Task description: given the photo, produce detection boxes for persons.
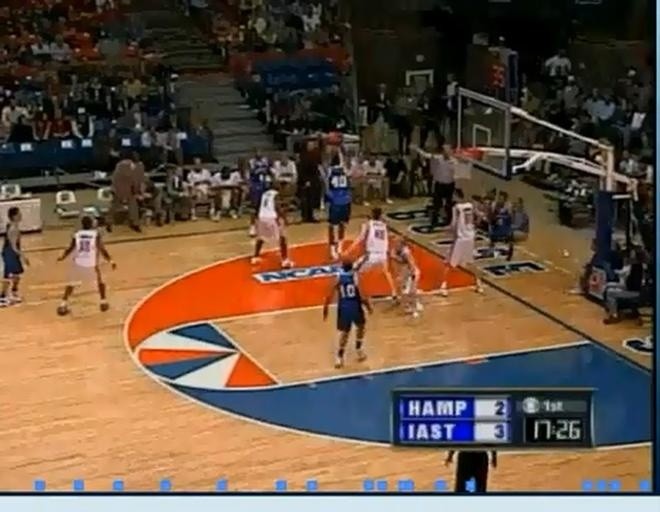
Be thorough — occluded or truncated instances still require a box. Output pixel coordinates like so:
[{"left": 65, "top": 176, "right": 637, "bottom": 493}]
[
  {"left": 0, "top": 0, "right": 657, "bottom": 369},
  {"left": 444, "top": 448, "right": 496, "bottom": 492}
]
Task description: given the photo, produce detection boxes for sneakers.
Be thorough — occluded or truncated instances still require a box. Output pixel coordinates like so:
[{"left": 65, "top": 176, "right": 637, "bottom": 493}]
[
  {"left": 362, "top": 200, "right": 370, "bottom": 207},
  {"left": 335, "top": 356, "right": 344, "bottom": 368},
  {"left": 571, "top": 287, "right": 586, "bottom": 294},
  {"left": 251, "top": 257, "right": 263, "bottom": 264},
  {"left": 470, "top": 284, "right": 484, "bottom": 293},
  {"left": 282, "top": 257, "right": 295, "bottom": 268},
  {"left": 329, "top": 249, "right": 343, "bottom": 259},
  {"left": 107, "top": 208, "right": 239, "bottom": 232},
  {"left": 603, "top": 314, "right": 621, "bottom": 325},
  {"left": 433, "top": 288, "right": 449, "bottom": 296},
  {"left": 249, "top": 225, "right": 257, "bottom": 236},
  {"left": 57, "top": 306, "right": 66, "bottom": 315},
  {"left": 0, "top": 294, "right": 22, "bottom": 306},
  {"left": 386, "top": 198, "right": 393, "bottom": 204},
  {"left": 100, "top": 302, "right": 110, "bottom": 310},
  {"left": 357, "top": 349, "right": 368, "bottom": 362}
]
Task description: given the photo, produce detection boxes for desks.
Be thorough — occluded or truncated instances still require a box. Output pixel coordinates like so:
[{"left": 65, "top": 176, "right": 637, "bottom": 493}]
[{"left": 0, "top": 198, "right": 41, "bottom": 234}]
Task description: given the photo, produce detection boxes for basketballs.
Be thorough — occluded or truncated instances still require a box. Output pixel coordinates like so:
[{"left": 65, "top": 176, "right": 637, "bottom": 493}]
[{"left": 328, "top": 133, "right": 342, "bottom": 145}]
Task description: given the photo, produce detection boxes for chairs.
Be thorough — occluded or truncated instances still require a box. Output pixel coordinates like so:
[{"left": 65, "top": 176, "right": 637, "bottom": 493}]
[
  {"left": 0, "top": 184, "right": 21, "bottom": 195},
  {"left": 53, "top": 190, "right": 80, "bottom": 230},
  {"left": 96, "top": 187, "right": 113, "bottom": 213}
]
